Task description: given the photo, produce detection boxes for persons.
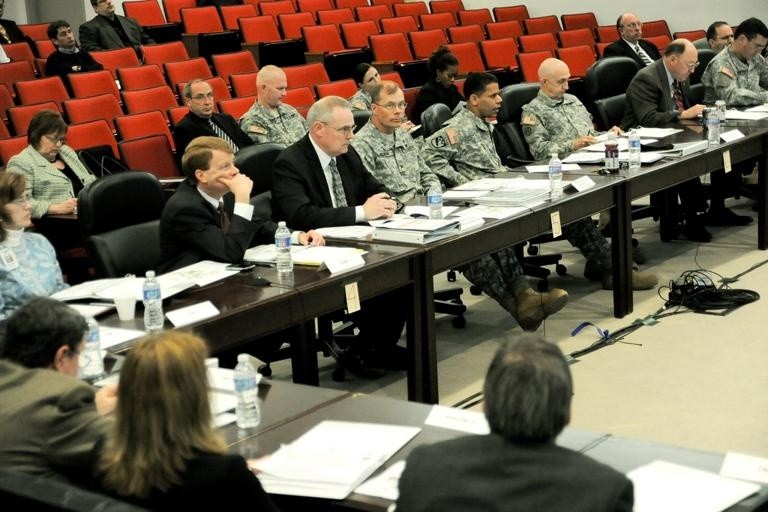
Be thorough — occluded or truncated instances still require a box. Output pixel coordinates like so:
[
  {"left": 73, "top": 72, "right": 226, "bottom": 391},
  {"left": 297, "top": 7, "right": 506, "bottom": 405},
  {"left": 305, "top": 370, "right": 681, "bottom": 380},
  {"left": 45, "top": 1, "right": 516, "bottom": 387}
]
[
  {"left": 0, "top": 1, "right": 24, "bottom": 64},
  {"left": 0, "top": 171, "right": 68, "bottom": 323},
  {"left": 411, "top": 47, "right": 464, "bottom": 124},
  {"left": 520, "top": 58, "right": 648, "bottom": 265},
  {"left": 172, "top": 79, "right": 256, "bottom": 176},
  {"left": 44, "top": 21, "right": 104, "bottom": 78},
  {"left": 158, "top": 136, "right": 325, "bottom": 276},
  {"left": 706, "top": 21, "right": 733, "bottom": 51},
  {"left": 351, "top": 80, "right": 568, "bottom": 333},
  {"left": 0, "top": 297, "right": 117, "bottom": 487},
  {"left": 92, "top": 332, "right": 278, "bottom": 512},
  {"left": 419, "top": 70, "right": 658, "bottom": 291},
  {"left": 4, "top": 111, "right": 98, "bottom": 220},
  {"left": 619, "top": 39, "right": 713, "bottom": 243},
  {"left": 603, "top": 13, "right": 662, "bottom": 69},
  {"left": 394, "top": 335, "right": 634, "bottom": 512},
  {"left": 701, "top": 18, "right": 768, "bottom": 107},
  {"left": 269, "top": 95, "right": 407, "bottom": 380},
  {"left": 79, "top": 0, "right": 157, "bottom": 51},
  {"left": 349, "top": 63, "right": 416, "bottom": 134},
  {"left": 237, "top": 65, "right": 308, "bottom": 149}
]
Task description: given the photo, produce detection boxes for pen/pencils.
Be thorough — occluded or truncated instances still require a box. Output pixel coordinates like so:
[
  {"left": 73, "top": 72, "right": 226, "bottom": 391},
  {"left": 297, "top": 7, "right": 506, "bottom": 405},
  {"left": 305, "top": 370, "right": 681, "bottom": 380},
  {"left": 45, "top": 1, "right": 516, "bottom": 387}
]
[
  {"left": 252, "top": 262, "right": 276, "bottom": 269},
  {"left": 586, "top": 129, "right": 591, "bottom": 137},
  {"left": 68, "top": 190, "right": 74, "bottom": 199},
  {"left": 382, "top": 196, "right": 401, "bottom": 200}
]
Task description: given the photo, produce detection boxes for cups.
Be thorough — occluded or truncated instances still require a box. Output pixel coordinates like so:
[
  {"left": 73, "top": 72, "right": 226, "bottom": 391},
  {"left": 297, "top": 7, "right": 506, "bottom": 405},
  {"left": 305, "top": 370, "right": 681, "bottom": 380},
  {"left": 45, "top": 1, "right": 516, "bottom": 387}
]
[{"left": 111, "top": 292, "right": 137, "bottom": 321}]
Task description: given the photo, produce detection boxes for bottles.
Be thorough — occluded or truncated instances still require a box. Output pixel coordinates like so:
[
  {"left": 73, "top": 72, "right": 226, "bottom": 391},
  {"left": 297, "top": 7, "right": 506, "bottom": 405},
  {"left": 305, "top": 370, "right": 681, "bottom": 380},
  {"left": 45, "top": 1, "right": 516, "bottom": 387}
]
[
  {"left": 628, "top": 129, "right": 641, "bottom": 168},
  {"left": 708, "top": 108, "right": 720, "bottom": 145},
  {"left": 548, "top": 153, "right": 563, "bottom": 197},
  {"left": 143, "top": 271, "right": 164, "bottom": 329},
  {"left": 604, "top": 143, "right": 619, "bottom": 173},
  {"left": 702, "top": 108, "right": 711, "bottom": 129},
  {"left": 80, "top": 317, "right": 104, "bottom": 375},
  {"left": 274, "top": 222, "right": 294, "bottom": 272},
  {"left": 715, "top": 100, "right": 726, "bottom": 122},
  {"left": 233, "top": 354, "right": 261, "bottom": 429},
  {"left": 426, "top": 182, "right": 443, "bottom": 220}
]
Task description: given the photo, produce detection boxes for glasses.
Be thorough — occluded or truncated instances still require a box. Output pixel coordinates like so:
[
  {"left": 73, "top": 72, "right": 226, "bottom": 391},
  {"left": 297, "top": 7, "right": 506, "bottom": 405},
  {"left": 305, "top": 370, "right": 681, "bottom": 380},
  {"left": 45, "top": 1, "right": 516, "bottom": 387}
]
[
  {"left": 43, "top": 134, "right": 67, "bottom": 145},
  {"left": 619, "top": 22, "right": 644, "bottom": 29},
  {"left": 191, "top": 93, "right": 214, "bottom": 100},
  {"left": 7, "top": 196, "right": 35, "bottom": 206},
  {"left": 373, "top": 101, "right": 407, "bottom": 110},
  {"left": 675, "top": 59, "right": 700, "bottom": 68},
  {"left": 321, "top": 121, "right": 357, "bottom": 135}
]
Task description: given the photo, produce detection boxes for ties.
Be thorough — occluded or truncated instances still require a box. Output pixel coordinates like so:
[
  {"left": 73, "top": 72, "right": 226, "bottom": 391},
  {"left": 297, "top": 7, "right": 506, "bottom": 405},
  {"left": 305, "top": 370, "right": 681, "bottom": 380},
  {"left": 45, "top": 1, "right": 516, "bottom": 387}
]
[
  {"left": 633, "top": 45, "right": 652, "bottom": 66},
  {"left": 205, "top": 117, "right": 240, "bottom": 156},
  {"left": 671, "top": 82, "right": 686, "bottom": 114},
  {"left": 329, "top": 160, "right": 350, "bottom": 210},
  {"left": 217, "top": 201, "right": 231, "bottom": 235}
]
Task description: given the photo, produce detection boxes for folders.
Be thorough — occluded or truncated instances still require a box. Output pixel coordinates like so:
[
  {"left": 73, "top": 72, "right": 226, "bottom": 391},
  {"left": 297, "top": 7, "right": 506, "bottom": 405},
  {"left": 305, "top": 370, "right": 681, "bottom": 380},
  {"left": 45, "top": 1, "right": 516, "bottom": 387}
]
[
  {"left": 471, "top": 186, "right": 552, "bottom": 207},
  {"left": 372, "top": 229, "right": 462, "bottom": 247},
  {"left": 97, "top": 325, "right": 147, "bottom": 355},
  {"left": 656, "top": 139, "right": 710, "bottom": 158},
  {"left": 375, "top": 219, "right": 462, "bottom": 234}
]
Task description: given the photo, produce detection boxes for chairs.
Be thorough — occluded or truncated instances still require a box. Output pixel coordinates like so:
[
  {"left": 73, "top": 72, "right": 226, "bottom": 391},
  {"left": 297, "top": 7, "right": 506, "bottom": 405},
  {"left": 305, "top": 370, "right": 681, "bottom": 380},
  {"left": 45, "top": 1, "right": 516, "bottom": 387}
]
[
  {"left": 493, "top": 5, "right": 530, "bottom": 31},
  {"left": 0, "top": 59, "right": 35, "bottom": 96},
  {"left": 523, "top": 15, "right": 562, "bottom": 44},
  {"left": 421, "top": 102, "right": 567, "bottom": 295},
  {"left": 0, "top": 134, "right": 31, "bottom": 163},
  {"left": 583, "top": 57, "right": 660, "bottom": 221},
  {"left": 161, "top": 0, "right": 196, "bottom": 24},
  {"left": 5, "top": 98, "right": 62, "bottom": 136},
  {"left": 122, "top": 0, "right": 176, "bottom": 32},
  {"left": 277, "top": 11, "right": 317, "bottom": 41},
  {"left": 162, "top": 56, "right": 231, "bottom": 95},
  {"left": 408, "top": 28, "right": 448, "bottom": 63},
  {"left": 116, "top": 64, "right": 178, "bottom": 100},
  {"left": 517, "top": 32, "right": 557, "bottom": 58},
  {"left": 220, "top": 3, "right": 258, "bottom": 31},
  {"left": 380, "top": 72, "right": 404, "bottom": 88},
  {"left": 484, "top": 20, "right": 524, "bottom": 47},
  {"left": 447, "top": 24, "right": 486, "bottom": 52},
  {"left": 498, "top": 82, "right": 639, "bottom": 255},
  {"left": 392, "top": 2, "right": 429, "bottom": 27},
  {"left": 33, "top": 57, "right": 50, "bottom": 76},
  {"left": 515, "top": 49, "right": 555, "bottom": 83},
  {"left": 2, "top": 42, "right": 37, "bottom": 72},
  {"left": 279, "top": 62, "right": 330, "bottom": 99},
  {"left": 368, "top": 32, "right": 425, "bottom": 68},
  {"left": 343, "top": 111, "right": 466, "bottom": 336},
  {"left": 335, "top": 0, "right": 369, "bottom": 18},
  {"left": 12, "top": 75, "right": 70, "bottom": 113},
  {"left": 113, "top": 109, "right": 178, "bottom": 155},
  {"left": 225, "top": 141, "right": 366, "bottom": 377},
  {"left": 60, "top": 92, "right": 124, "bottom": 136},
  {"left": 480, "top": 37, "right": 519, "bottom": 72},
  {"left": 371, "top": 0, "right": 404, "bottom": 17},
  {"left": 445, "top": 41, "right": 503, "bottom": 77},
  {"left": 340, "top": 19, "right": 380, "bottom": 51},
  {"left": 641, "top": 20, "right": 673, "bottom": 41},
  {"left": 429, "top": 0, "right": 465, "bottom": 25},
  {"left": 117, "top": 132, "right": 186, "bottom": 190},
  {"left": 167, "top": 107, "right": 189, "bottom": 128},
  {"left": 18, "top": 21, "right": 50, "bottom": 41},
  {"left": 417, "top": 11, "right": 457, "bottom": 42},
  {"left": 78, "top": 171, "right": 165, "bottom": 280},
  {"left": 297, "top": 0, "right": 333, "bottom": 22},
  {"left": 315, "top": 7, "right": 355, "bottom": 34},
  {"left": 594, "top": 25, "right": 621, "bottom": 42},
  {"left": 314, "top": 78, "right": 358, "bottom": 103},
  {"left": 237, "top": 14, "right": 297, "bottom": 47},
  {"left": 67, "top": 68, "right": 123, "bottom": 105},
  {"left": 0, "top": 83, "right": 16, "bottom": 123},
  {"left": 594, "top": 42, "right": 613, "bottom": 59},
  {"left": 229, "top": 71, "right": 258, "bottom": 99},
  {"left": 457, "top": 8, "right": 494, "bottom": 35},
  {"left": 687, "top": 49, "right": 759, "bottom": 212},
  {"left": 35, "top": 39, "right": 57, "bottom": 59},
  {"left": 673, "top": 30, "right": 706, "bottom": 43},
  {"left": 139, "top": 40, "right": 190, "bottom": 78},
  {"left": 179, "top": 5, "right": 234, "bottom": 37},
  {"left": 301, "top": 22, "right": 364, "bottom": 56},
  {"left": 642, "top": 35, "right": 670, "bottom": 56},
  {"left": 88, "top": 47, "right": 140, "bottom": 82},
  {"left": 60, "top": 118, "right": 121, "bottom": 161},
  {"left": 216, "top": 93, "right": 257, "bottom": 119},
  {"left": 258, "top": 0, "right": 296, "bottom": 25},
  {"left": 281, "top": 86, "right": 316, "bottom": 118},
  {"left": 175, "top": 76, "right": 232, "bottom": 115},
  {"left": 379, "top": 14, "right": 418, "bottom": 44},
  {"left": 402, "top": 89, "right": 420, "bottom": 116},
  {"left": 557, "top": 45, "right": 596, "bottom": 78},
  {"left": 354, "top": 4, "right": 392, "bottom": 33},
  {"left": 556, "top": 28, "right": 597, "bottom": 58},
  {"left": 561, "top": 12, "right": 598, "bottom": 40},
  {"left": 211, "top": 49, "right": 259, "bottom": 88},
  {"left": 120, "top": 83, "right": 179, "bottom": 126}
]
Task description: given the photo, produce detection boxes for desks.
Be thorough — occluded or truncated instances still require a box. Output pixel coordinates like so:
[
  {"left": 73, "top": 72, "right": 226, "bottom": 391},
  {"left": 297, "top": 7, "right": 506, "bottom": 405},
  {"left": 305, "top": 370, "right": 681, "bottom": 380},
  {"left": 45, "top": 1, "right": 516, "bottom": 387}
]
[
  {"left": 663, "top": 103, "right": 768, "bottom": 128},
  {"left": 103, "top": 353, "right": 351, "bottom": 452},
  {"left": 95, "top": 239, "right": 424, "bottom": 404},
  {"left": 357, "top": 172, "right": 628, "bottom": 404},
  {"left": 507, "top": 125, "right": 768, "bottom": 314},
  {"left": 222, "top": 392, "right": 768, "bottom": 512}
]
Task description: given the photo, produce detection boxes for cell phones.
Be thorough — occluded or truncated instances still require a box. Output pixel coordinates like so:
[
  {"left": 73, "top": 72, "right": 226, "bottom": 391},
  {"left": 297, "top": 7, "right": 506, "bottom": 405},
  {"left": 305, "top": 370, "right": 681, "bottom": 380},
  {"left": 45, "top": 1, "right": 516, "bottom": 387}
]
[{"left": 226, "top": 262, "right": 255, "bottom": 269}]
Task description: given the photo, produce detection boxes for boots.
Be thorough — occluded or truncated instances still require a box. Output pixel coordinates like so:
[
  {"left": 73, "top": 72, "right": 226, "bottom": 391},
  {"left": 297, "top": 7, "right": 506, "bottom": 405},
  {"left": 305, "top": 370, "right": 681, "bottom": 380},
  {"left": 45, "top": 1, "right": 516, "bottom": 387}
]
[
  {"left": 499, "top": 295, "right": 542, "bottom": 333},
  {"left": 507, "top": 276, "right": 569, "bottom": 329},
  {"left": 603, "top": 268, "right": 659, "bottom": 290}
]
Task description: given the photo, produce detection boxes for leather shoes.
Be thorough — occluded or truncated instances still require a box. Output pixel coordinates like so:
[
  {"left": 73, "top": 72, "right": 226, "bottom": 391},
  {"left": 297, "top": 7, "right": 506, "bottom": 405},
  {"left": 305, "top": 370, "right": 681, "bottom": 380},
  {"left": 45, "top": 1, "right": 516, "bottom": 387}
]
[
  {"left": 706, "top": 207, "right": 754, "bottom": 227},
  {"left": 678, "top": 215, "right": 713, "bottom": 243}
]
